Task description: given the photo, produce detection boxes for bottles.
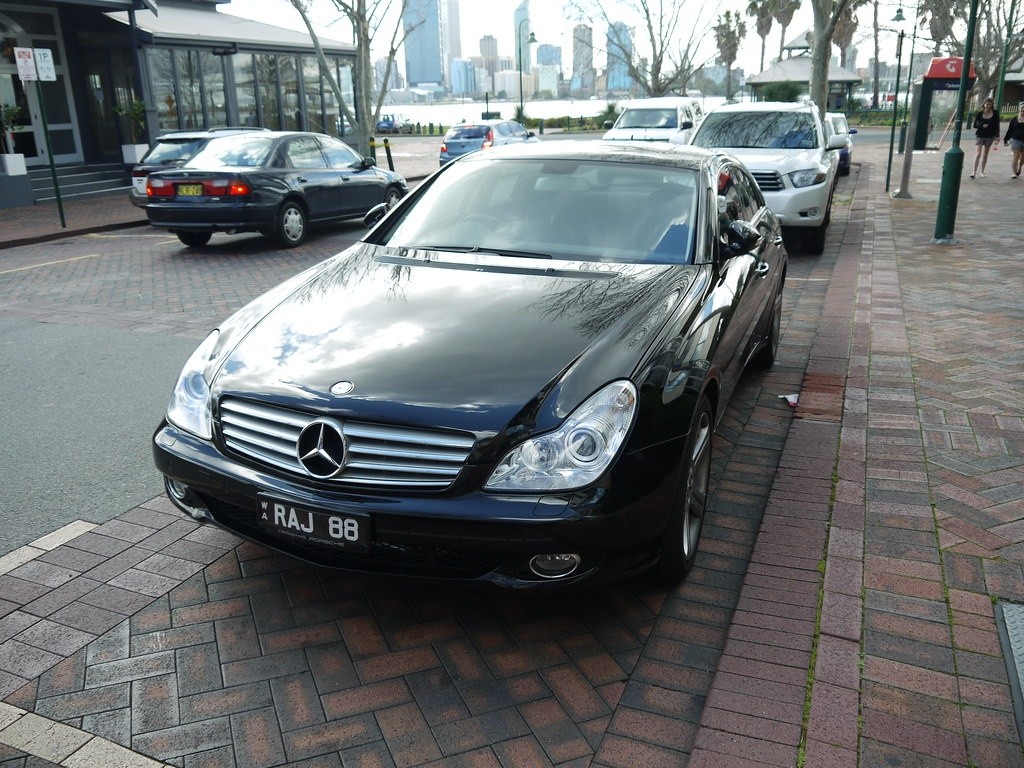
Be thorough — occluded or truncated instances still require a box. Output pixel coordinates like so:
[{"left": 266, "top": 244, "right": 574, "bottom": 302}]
[{"left": 994, "top": 141, "right": 998, "bottom": 152}]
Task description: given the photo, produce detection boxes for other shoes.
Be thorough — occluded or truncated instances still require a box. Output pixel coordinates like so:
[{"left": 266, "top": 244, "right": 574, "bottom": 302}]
[
  {"left": 980, "top": 173, "right": 987, "bottom": 177},
  {"left": 1017, "top": 171, "right": 1021, "bottom": 176},
  {"left": 1011, "top": 174, "right": 1017, "bottom": 178},
  {"left": 970, "top": 175, "right": 975, "bottom": 179}
]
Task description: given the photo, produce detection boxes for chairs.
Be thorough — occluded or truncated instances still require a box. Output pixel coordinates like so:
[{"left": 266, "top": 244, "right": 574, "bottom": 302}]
[
  {"left": 627, "top": 184, "right": 694, "bottom": 254},
  {"left": 487, "top": 190, "right": 573, "bottom": 245}
]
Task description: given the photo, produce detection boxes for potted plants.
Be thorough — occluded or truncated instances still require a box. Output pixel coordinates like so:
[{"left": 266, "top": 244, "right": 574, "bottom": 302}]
[
  {"left": 111, "top": 96, "right": 150, "bottom": 165},
  {"left": 0, "top": 104, "right": 31, "bottom": 176}
]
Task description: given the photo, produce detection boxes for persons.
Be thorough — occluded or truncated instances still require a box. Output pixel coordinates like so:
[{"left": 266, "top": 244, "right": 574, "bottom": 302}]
[
  {"left": 1003, "top": 102, "right": 1024, "bottom": 178},
  {"left": 970, "top": 98, "right": 1001, "bottom": 179}
]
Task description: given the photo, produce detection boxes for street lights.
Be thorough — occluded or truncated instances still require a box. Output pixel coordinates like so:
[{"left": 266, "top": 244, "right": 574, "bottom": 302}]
[
  {"left": 891, "top": 0, "right": 921, "bottom": 156},
  {"left": 519, "top": 18, "right": 539, "bottom": 125}
]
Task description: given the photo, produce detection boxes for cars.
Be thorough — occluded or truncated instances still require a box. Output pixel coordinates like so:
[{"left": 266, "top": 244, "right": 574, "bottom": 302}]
[
  {"left": 823, "top": 111, "right": 859, "bottom": 175},
  {"left": 128, "top": 127, "right": 273, "bottom": 208},
  {"left": 376, "top": 113, "right": 414, "bottom": 134},
  {"left": 439, "top": 120, "right": 542, "bottom": 168},
  {"left": 146, "top": 130, "right": 411, "bottom": 249},
  {"left": 152, "top": 139, "right": 790, "bottom": 599}
]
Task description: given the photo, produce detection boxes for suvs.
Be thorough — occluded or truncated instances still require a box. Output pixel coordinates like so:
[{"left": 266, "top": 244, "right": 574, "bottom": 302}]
[
  {"left": 684, "top": 100, "right": 849, "bottom": 256},
  {"left": 602, "top": 98, "right": 704, "bottom": 148}
]
